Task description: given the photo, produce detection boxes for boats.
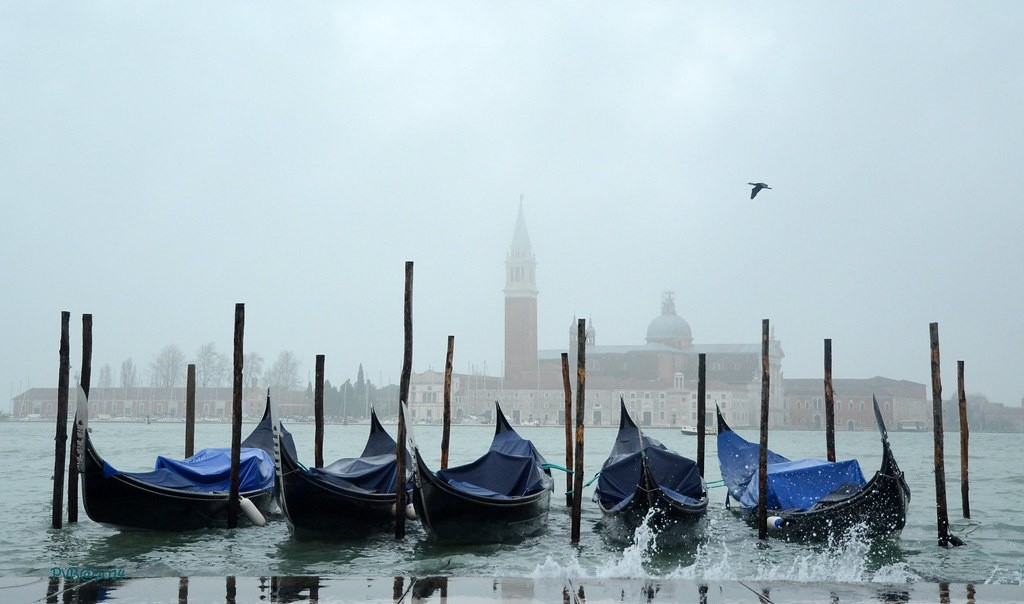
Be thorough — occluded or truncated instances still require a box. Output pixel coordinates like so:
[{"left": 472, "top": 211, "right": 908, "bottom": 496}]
[
  {"left": 715, "top": 405, "right": 911, "bottom": 545},
  {"left": 595, "top": 396, "right": 708, "bottom": 527},
  {"left": 75, "top": 379, "right": 279, "bottom": 531},
  {"left": 270, "top": 389, "right": 414, "bottom": 534},
  {"left": 681, "top": 424, "right": 716, "bottom": 437},
  {"left": 397, "top": 400, "right": 555, "bottom": 542}
]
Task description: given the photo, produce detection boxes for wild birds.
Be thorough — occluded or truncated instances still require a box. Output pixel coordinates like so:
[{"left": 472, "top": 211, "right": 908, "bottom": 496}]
[{"left": 747, "top": 182, "right": 772, "bottom": 200}]
